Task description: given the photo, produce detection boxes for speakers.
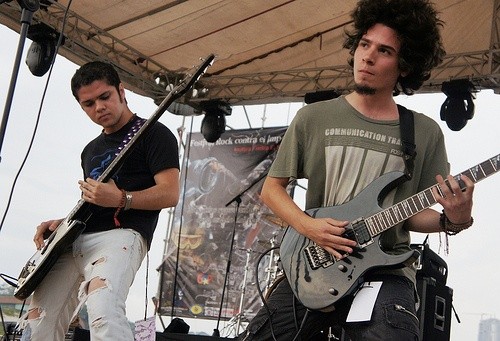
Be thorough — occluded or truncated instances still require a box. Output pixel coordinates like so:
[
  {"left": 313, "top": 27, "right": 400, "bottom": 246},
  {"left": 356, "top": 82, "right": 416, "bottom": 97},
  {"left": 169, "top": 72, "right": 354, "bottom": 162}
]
[{"left": 414, "top": 276, "right": 453, "bottom": 341}]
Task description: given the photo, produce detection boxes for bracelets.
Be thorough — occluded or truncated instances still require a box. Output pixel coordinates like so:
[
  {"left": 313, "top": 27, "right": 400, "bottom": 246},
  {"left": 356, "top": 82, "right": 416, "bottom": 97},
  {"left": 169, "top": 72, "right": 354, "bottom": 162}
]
[
  {"left": 439, "top": 210, "right": 474, "bottom": 236},
  {"left": 114, "top": 189, "right": 125, "bottom": 226},
  {"left": 123, "top": 192, "right": 132, "bottom": 210}
]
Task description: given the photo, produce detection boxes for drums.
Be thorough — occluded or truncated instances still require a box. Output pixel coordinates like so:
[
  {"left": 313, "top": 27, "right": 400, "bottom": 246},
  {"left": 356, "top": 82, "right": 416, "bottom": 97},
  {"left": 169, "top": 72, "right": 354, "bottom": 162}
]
[
  {"left": 274, "top": 256, "right": 285, "bottom": 281},
  {"left": 261, "top": 274, "right": 286, "bottom": 307}
]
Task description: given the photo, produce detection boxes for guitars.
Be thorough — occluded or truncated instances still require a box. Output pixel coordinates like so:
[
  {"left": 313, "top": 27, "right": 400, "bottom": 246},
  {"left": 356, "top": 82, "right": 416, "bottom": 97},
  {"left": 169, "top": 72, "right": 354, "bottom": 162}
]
[
  {"left": 13, "top": 52, "right": 215, "bottom": 302},
  {"left": 280, "top": 152, "right": 500, "bottom": 314}
]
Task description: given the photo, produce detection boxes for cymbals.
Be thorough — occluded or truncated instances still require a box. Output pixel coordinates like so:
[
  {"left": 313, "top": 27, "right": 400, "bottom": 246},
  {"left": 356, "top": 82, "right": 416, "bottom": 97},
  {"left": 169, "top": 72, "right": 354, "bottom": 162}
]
[
  {"left": 257, "top": 240, "right": 280, "bottom": 256},
  {"left": 263, "top": 214, "right": 290, "bottom": 229}
]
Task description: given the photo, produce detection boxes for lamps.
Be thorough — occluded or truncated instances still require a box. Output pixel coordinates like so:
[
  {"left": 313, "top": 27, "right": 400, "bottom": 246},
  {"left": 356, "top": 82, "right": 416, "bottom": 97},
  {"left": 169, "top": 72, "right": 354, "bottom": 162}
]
[
  {"left": 25, "top": 22, "right": 65, "bottom": 77},
  {"left": 152, "top": 71, "right": 232, "bottom": 143},
  {"left": 440, "top": 76, "right": 476, "bottom": 131}
]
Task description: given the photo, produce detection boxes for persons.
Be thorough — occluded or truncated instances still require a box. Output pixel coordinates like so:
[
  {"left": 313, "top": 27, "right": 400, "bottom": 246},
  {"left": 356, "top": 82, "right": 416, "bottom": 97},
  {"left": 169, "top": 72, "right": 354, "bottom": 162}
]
[
  {"left": 15, "top": 62, "right": 180, "bottom": 341},
  {"left": 236, "top": 0, "right": 474, "bottom": 341}
]
